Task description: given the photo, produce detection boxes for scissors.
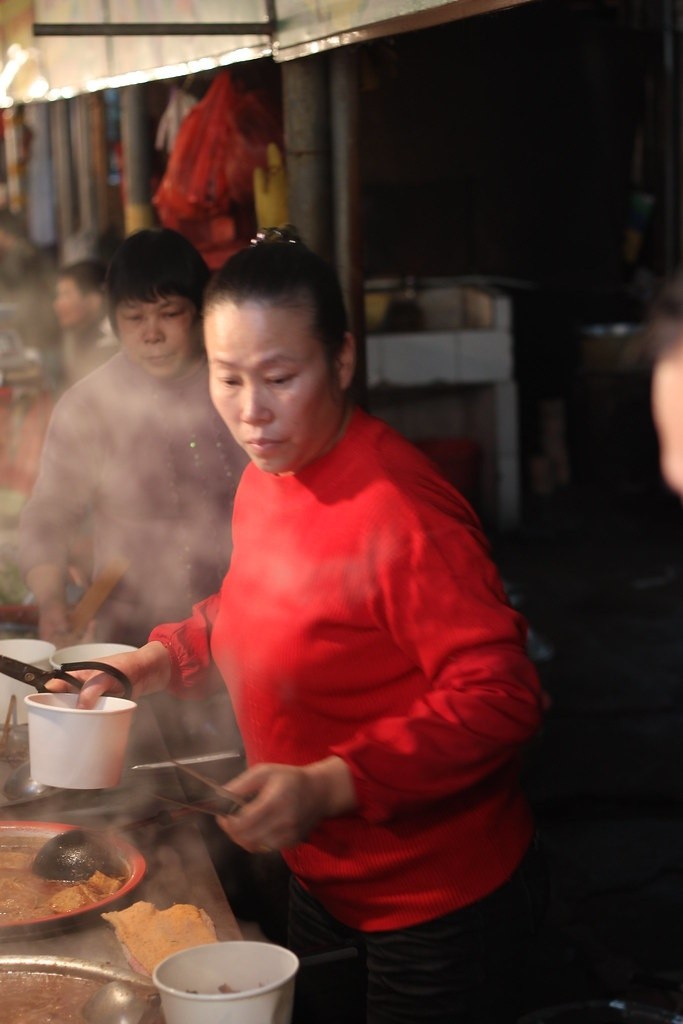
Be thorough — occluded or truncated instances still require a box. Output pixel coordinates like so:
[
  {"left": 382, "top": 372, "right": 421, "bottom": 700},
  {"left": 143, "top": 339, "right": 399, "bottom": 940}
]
[{"left": 0, "top": 655, "right": 134, "bottom": 700}]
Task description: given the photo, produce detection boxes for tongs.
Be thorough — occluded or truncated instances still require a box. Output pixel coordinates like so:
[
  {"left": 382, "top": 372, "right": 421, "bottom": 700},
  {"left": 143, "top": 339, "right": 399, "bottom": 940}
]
[{"left": 133, "top": 745, "right": 323, "bottom": 852}]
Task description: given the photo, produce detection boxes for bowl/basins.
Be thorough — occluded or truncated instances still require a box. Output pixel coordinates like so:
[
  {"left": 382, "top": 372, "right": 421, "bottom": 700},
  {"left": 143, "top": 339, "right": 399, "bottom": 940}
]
[
  {"left": 49, "top": 642, "right": 139, "bottom": 673},
  {"left": 0, "top": 638, "right": 57, "bottom": 725},
  {"left": 24, "top": 691, "right": 137, "bottom": 790},
  {"left": 151, "top": 941, "right": 300, "bottom": 1024}
]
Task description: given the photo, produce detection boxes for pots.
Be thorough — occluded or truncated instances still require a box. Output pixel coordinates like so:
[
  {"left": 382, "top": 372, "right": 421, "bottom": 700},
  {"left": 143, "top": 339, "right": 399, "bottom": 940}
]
[
  {"left": 0, "top": 721, "right": 110, "bottom": 809},
  {"left": 0, "top": 952, "right": 185, "bottom": 1024},
  {"left": 0, "top": 815, "right": 149, "bottom": 942}
]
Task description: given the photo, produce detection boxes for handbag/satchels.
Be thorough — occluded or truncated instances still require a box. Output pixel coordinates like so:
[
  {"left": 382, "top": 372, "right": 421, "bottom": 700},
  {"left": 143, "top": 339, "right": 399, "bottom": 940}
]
[{"left": 153, "top": 71, "right": 275, "bottom": 231}]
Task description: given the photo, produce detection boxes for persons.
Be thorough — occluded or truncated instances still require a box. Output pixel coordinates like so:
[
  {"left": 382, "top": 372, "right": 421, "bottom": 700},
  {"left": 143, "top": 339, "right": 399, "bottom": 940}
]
[
  {"left": 42, "top": 226, "right": 550, "bottom": 1024},
  {"left": 32, "top": 229, "right": 233, "bottom": 649},
  {"left": 54, "top": 263, "right": 106, "bottom": 368}
]
